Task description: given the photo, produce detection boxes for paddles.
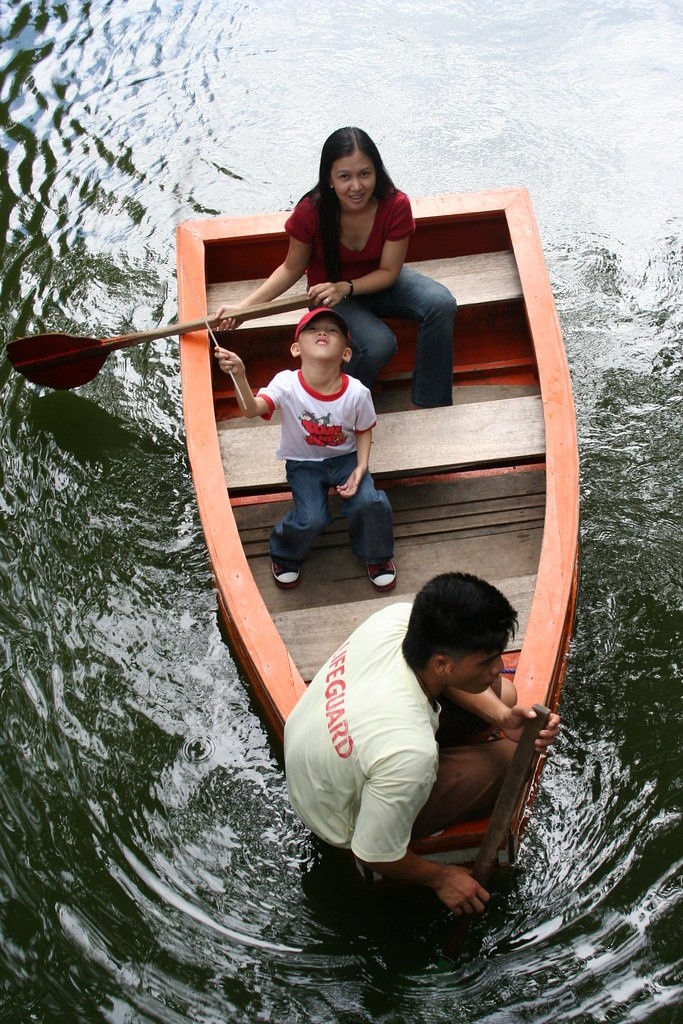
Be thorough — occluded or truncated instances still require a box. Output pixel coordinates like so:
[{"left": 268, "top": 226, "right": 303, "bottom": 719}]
[
  {"left": 439, "top": 700, "right": 554, "bottom": 962},
  {"left": 4, "top": 291, "right": 319, "bottom": 393}
]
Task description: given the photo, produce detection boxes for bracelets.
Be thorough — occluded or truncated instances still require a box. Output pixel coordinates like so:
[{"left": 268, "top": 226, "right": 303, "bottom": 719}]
[{"left": 346, "top": 279, "right": 353, "bottom": 297}]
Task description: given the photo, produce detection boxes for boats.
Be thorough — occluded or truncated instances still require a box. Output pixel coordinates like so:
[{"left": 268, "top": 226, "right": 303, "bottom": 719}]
[{"left": 176, "top": 186, "right": 581, "bottom": 900}]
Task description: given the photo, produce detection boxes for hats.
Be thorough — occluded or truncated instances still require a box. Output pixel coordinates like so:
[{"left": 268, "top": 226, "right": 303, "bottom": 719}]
[{"left": 295, "top": 307, "right": 350, "bottom": 348}]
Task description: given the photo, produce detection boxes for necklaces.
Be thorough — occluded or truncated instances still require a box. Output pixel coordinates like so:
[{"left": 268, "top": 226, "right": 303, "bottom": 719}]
[{"left": 415, "top": 671, "right": 437, "bottom": 705}]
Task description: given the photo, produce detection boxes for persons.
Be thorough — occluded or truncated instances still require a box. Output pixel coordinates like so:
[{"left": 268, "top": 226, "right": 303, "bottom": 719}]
[
  {"left": 213, "top": 127, "right": 457, "bottom": 408},
  {"left": 214, "top": 308, "right": 397, "bottom": 592},
  {"left": 283, "top": 573, "right": 562, "bottom": 914}
]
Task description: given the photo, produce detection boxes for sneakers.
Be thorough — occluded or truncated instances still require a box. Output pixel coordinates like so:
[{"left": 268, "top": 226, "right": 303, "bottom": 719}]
[
  {"left": 366, "top": 559, "right": 396, "bottom": 591},
  {"left": 272, "top": 560, "right": 300, "bottom": 588}
]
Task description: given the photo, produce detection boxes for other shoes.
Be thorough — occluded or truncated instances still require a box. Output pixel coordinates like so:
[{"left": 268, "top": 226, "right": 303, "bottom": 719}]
[{"left": 407, "top": 401, "right": 425, "bottom": 411}]
